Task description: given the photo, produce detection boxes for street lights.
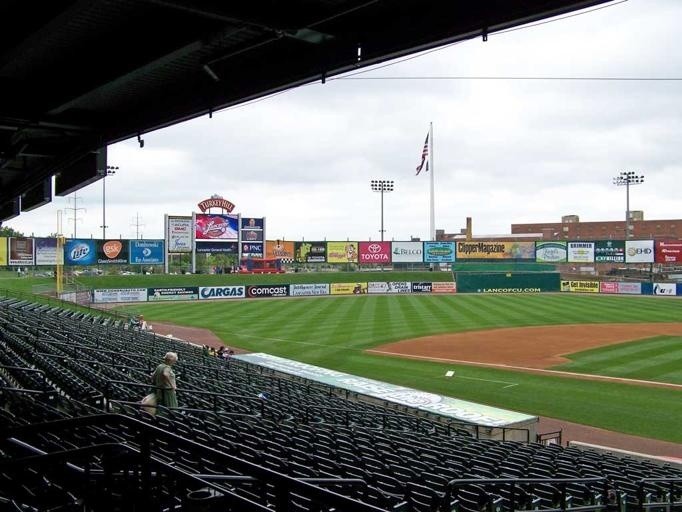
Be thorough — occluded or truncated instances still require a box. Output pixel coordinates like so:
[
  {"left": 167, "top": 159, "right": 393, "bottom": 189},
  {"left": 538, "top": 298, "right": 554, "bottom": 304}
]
[
  {"left": 100, "top": 166, "right": 119, "bottom": 239},
  {"left": 613, "top": 171, "right": 644, "bottom": 239},
  {"left": 370, "top": 180, "right": 394, "bottom": 241}
]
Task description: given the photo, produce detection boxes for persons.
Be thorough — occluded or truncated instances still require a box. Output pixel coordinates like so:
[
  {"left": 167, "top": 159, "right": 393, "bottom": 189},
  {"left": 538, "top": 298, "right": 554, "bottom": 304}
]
[
  {"left": 202, "top": 344, "right": 234, "bottom": 361},
  {"left": 130, "top": 314, "right": 144, "bottom": 328},
  {"left": 150, "top": 352, "right": 178, "bottom": 416}
]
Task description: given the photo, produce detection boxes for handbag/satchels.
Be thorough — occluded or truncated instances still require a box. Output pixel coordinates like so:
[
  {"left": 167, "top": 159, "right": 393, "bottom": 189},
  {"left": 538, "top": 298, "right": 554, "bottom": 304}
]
[{"left": 141, "top": 393, "right": 158, "bottom": 415}]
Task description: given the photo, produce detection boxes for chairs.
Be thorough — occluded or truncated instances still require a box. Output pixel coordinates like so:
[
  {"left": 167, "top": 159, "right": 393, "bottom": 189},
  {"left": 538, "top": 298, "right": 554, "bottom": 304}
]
[{"left": 0, "top": 299, "right": 682, "bottom": 511}]
